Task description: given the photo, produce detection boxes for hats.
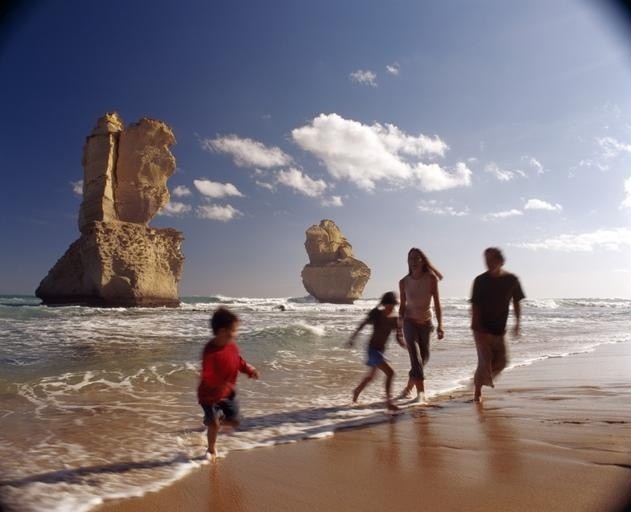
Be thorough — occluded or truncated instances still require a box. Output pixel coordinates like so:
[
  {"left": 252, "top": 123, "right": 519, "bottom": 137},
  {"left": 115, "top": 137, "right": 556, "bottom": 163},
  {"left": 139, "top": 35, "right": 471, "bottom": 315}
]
[{"left": 381, "top": 292, "right": 398, "bottom": 304}]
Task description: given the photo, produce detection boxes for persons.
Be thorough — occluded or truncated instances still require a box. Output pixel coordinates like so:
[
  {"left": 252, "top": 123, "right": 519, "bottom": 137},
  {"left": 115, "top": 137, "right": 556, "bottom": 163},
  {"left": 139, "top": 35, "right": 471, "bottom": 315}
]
[
  {"left": 470, "top": 247, "right": 527, "bottom": 402},
  {"left": 197, "top": 308, "right": 261, "bottom": 463},
  {"left": 344, "top": 247, "right": 445, "bottom": 410}
]
